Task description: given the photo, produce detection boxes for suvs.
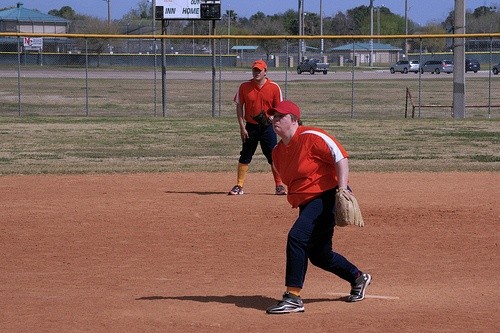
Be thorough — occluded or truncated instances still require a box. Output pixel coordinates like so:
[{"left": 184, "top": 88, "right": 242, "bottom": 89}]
[{"left": 465, "top": 58, "right": 480, "bottom": 73}]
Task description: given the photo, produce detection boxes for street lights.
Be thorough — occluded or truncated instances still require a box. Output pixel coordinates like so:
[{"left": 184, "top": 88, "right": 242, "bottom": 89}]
[{"left": 374, "top": 4, "right": 385, "bottom": 43}]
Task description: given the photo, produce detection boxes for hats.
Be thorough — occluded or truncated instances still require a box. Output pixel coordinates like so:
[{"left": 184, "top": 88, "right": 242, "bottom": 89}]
[
  {"left": 267, "top": 101, "right": 300, "bottom": 118},
  {"left": 252, "top": 60, "right": 267, "bottom": 70}
]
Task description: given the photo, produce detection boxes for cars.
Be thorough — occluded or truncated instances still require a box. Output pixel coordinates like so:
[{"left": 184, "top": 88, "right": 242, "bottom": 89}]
[{"left": 493, "top": 63, "right": 500, "bottom": 75}]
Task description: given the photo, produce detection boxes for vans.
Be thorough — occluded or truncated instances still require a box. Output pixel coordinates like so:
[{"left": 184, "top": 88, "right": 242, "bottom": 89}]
[
  {"left": 390, "top": 59, "right": 420, "bottom": 74},
  {"left": 420, "top": 59, "right": 453, "bottom": 74}
]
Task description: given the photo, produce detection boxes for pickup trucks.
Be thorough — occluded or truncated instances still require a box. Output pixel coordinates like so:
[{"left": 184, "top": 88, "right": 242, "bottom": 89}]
[{"left": 297, "top": 57, "right": 330, "bottom": 75}]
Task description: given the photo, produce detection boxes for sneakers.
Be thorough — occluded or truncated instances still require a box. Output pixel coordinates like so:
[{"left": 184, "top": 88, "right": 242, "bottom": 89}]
[
  {"left": 228, "top": 185, "right": 244, "bottom": 195},
  {"left": 266, "top": 291, "right": 304, "bottom": 313},
  {"left": 348, "top": 271, "right": 371, "bottom": 301},
  {"left": 276, "top": 186, "right": 287, "bottom": 195}
]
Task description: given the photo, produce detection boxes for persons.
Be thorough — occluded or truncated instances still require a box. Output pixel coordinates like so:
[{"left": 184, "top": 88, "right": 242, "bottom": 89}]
[
  {"left": 266, "top": 101, "right": 373, "bottom": 313},
  {"left": 228, "top": 60, "right": 289, "bottom": 195}
]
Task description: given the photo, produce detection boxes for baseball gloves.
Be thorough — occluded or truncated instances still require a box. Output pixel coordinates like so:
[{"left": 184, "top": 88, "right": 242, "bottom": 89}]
[
  {"left": 249, "top": 109, "right": 272, "bottom": 126},
  {"left": 336, "top": 189, "right": 364, "bottom": 228}
]
